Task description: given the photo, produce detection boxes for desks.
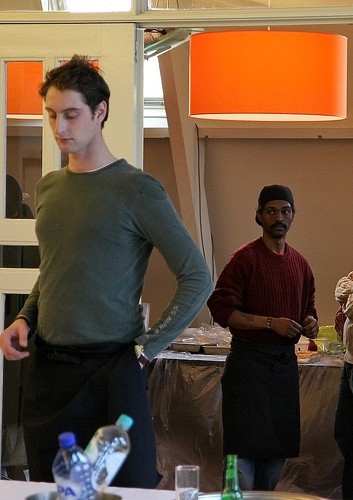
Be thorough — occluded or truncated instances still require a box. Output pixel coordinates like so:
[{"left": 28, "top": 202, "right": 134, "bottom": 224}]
[{"left": 130, "top": 336, "right": 348, "bottom": 500}]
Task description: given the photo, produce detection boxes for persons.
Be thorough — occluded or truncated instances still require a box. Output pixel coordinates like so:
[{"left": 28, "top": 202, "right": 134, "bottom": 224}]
[
  {"left": 335, "top": 272, "right": 353, "bottom": 500},
  {"left": 1, "top": 55, "right": 212, "bottom": 490},
  {"left": 207, "top": 185, "right": 319, "bottom": 492}
]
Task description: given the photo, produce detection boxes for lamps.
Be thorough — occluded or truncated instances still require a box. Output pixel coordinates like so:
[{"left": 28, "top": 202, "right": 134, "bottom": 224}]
[
  {"left": 188, "top": 0, "right": 348, "bottom": 122},
  {"left": 7, "top": 60, "right": 99, "bottom": 118}
]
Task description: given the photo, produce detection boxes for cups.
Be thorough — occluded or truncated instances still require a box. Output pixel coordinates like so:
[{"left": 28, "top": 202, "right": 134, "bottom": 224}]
[{"left": 175, "top": 464, "right": 200, "bottom": 500}]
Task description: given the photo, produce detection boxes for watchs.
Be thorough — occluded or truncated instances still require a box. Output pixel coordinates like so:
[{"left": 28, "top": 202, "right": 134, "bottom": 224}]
[{"left": 134, "top": 344, "right": 148, "bottom": 365}]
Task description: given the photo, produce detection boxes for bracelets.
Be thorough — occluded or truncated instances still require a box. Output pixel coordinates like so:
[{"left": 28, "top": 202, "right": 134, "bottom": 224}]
[
  {"left": 15, "top": 316, "right": 31, "bottom": 329},
  {"left": 267, "top": 317, "right": 272, "bottom": 329}
]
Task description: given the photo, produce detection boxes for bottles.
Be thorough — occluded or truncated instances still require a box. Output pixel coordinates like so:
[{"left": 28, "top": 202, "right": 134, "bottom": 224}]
[
  {"left": 220, "top": 454, "right": 243, "bottom": 500},
  {"left": 52, "top": 432, "right": 98, "bottom": 500},
  {"left": 83, "top": 414, "right": 133, "bottom": 495}
]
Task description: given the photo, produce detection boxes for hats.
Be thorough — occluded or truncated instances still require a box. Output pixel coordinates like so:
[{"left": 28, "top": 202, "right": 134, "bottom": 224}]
[{"left": 256, "top": 184, "right": 295, "bottom": 226}]
